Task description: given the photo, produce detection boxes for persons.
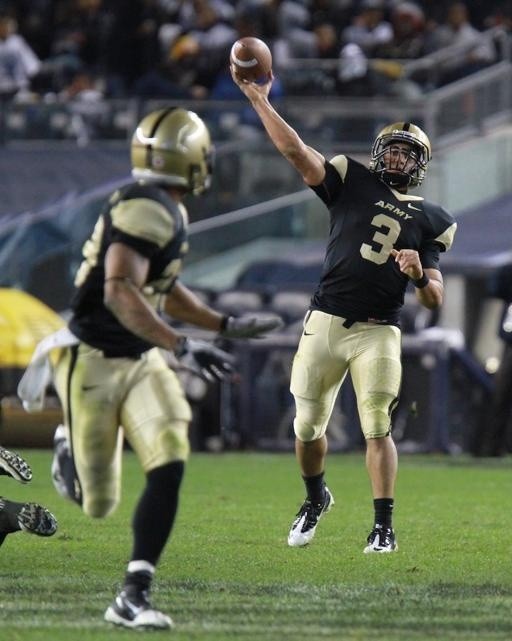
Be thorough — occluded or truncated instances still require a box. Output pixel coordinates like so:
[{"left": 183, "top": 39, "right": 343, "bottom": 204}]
[
  {"left": 226, "top": 62, "right": 457, "bottom": 555},
  {"left": 0, "top": 446, "right": 58, "bottom": 547},
  {"left": 49, "top": 110, "right": 285, "bottom": 632},
  {"left": 1, "top": 0, "right": 510, "bottom": 153}
]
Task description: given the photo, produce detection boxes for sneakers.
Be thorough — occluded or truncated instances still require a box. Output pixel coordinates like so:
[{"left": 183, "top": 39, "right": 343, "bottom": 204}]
[
  {"left": 287, "top": 487, "right": 334, "bottom": 547},
  {"left": 364, "top": 525, "right": 397, "bottom": 553},
  {"left": 1, "top": 423, "right": 176, "bottom": 631}
]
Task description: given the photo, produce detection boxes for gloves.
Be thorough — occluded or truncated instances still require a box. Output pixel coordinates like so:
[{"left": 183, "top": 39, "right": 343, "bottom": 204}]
[
  {"left": 173, "top": 337, "right": 236, "bottom": 383},
  {"left": 222, "top": 315, "right": 284, "bottom": 338}
]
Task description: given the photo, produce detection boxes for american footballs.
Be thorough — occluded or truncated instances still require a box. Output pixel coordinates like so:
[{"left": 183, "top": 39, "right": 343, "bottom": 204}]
[{"left": 232, "top": 36, "right": 272, "bottom": 81}]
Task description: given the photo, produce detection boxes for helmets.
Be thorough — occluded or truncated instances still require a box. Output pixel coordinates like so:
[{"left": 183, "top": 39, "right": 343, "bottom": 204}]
[
  {"left": 129, "top": 107, "right": 215, "bottom": 198},
  {"left": 369, "top": 122, "right": 434, "bottom": 188}
]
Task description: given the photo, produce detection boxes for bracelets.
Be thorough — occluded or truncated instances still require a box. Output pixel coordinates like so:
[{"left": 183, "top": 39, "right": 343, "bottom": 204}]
[
  {"left": 411, "top": 270, "right": 429, "bottom": 289},
  {"left": 218, "top": 312, "right": 230, "bottom": 336}
]
